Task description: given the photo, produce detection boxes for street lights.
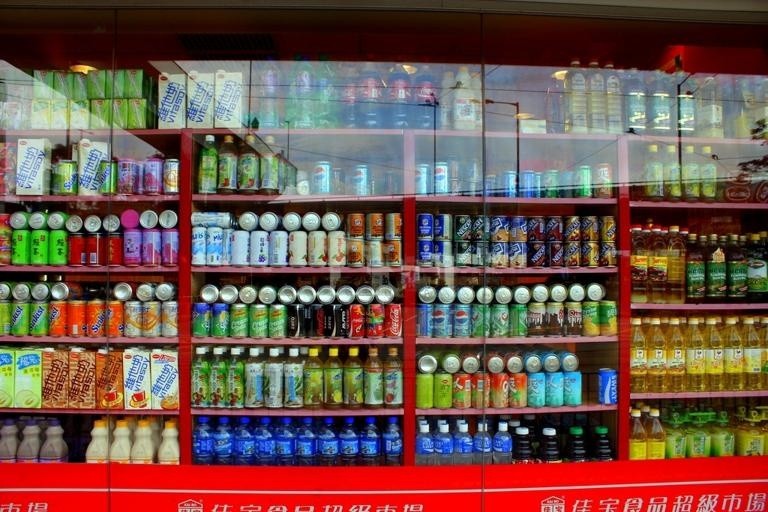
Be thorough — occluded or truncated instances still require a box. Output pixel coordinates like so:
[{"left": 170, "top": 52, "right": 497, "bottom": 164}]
[
  {"left": 485, "top": 96, "right": 521, "bottom": 198},
  {"left": 283, "top": 120, "right": 291, "bottom": 160},
  {"left": 677, "top": 90, "right": 695, "bottom": 198}
]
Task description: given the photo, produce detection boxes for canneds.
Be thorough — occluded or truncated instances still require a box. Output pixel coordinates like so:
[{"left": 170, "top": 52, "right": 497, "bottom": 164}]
[
  {"left": 0, "top": 158, "right": 179, "bottom": 339},
  {"left": 191, "top": 161, "right": 403, "bottom": 340},
  {"left": 415, "top": 155, "right": 613, "bottom": 198},
  {"left": 416, "top": 212, "right": 618, "bottom": 268},
  {"left": 415, "top": 349, "right": 583, "bottom": 407},
  {"left": 417, "top": 283, "right": 618, "bottom": 339},
  {"left": 598, "top": 368, "right": 618, "bottom": 404}
]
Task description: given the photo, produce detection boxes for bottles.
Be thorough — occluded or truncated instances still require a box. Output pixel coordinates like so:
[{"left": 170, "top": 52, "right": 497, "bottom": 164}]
[
  {"left": 415, "top": 412, "right": 612, "bottom": 465},
  {"left": 191, "top": 345, "right": 403, "bottom": 467},
  {"left": 197, "top": 135, "right": 296, "bottom": 196},
  {"left": 645, "top": 145, "right": 717, "bottom": 202},
  {"left": 255, "top": 48, "right": 480, "bottom": 133},
  {"left": 541, "top": 58, "right": 768, "bottom": 140},
  {"left": 628, "top": 219, "right": 768, "bottom": 461},
  {"left": 0, "top": 412, "right": 181, "bottom": 464}
]
